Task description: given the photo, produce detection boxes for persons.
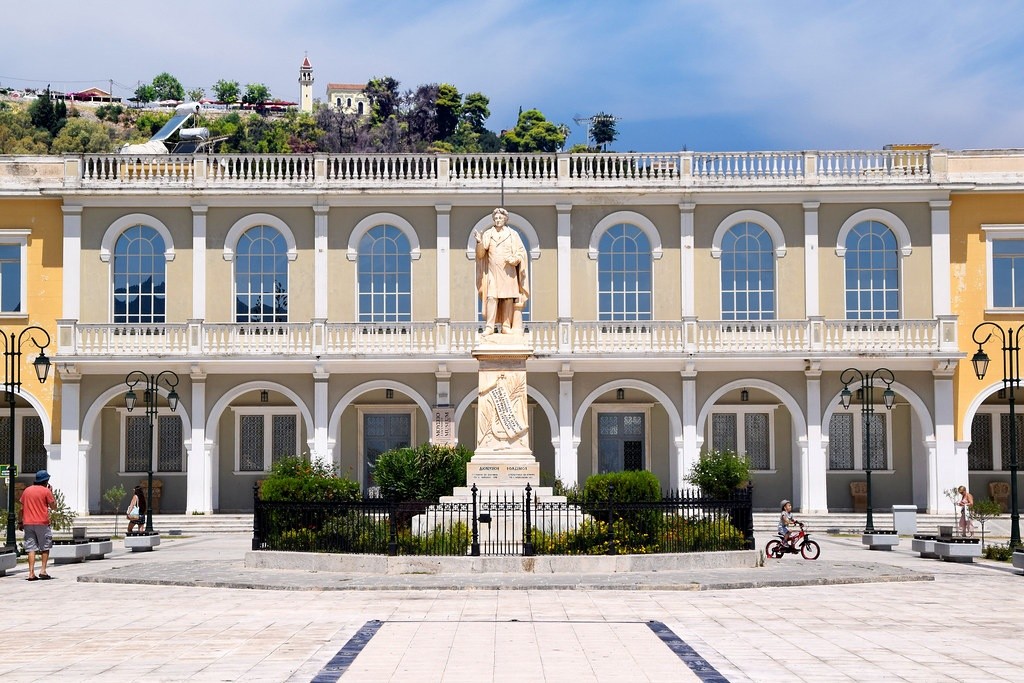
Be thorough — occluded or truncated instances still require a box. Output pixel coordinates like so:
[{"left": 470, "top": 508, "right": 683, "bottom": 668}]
[
  {"left": 957, "top": 487, "right": 974, "bottom": 538},
  {"left": 126, "top": 486, "right": 146, "bottom": 536},
  {"left": 17, "top": 470, "right": 57, "bottom": 581},
  {"left": 473, "top": 208, "right": 529, "bottom": 336},
  {"left": 778, "top": 500, "right": 804, "bottom": 551}
]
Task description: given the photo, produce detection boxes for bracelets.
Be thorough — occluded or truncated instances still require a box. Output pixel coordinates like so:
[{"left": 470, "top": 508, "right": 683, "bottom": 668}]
[{"left": 17, "top": 522, "right": 23, "bottom": 525}]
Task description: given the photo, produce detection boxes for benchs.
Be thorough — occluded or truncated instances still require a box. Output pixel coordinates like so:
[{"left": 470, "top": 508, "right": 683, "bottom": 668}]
[
  {"left": 912, "top": 534, "right": 940, "bottom": 558},
  {"left": 49, "top": 538, "right": 92, "bottom": 564},
  {"left": 1013, "top": 548, "right": 1024, "bottom": 570},
  {"left": 0, "top": 546, "right": 16, "bottom": 577},
  {"left": 862, "top": 530, "right": 899, "bottom": 550},
  {"left": 934, "top": 538, "right": 982, "bottom": 562},
  {"left": 124, "top": 531, "right": 160, "bottom": 552},
  {"left": 71, "top": 537, "right": 112, "bottom": 560}
]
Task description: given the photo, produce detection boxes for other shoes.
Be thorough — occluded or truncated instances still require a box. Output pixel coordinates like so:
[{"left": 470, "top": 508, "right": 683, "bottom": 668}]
[{"left": 781, "top": 541, "right": 789, "bottom": 548}]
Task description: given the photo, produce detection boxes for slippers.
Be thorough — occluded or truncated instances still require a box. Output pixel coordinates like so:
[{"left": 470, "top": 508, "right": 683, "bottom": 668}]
[
  {"left": 28, "top": 576, "right": 38, "bottom": 581},
  {"left": 39, "top": 573, "right": 51, "bottom": 579}
]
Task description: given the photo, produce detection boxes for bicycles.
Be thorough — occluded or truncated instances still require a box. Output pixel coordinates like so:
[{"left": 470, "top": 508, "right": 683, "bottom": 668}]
[{"left": 766, "top": 520, "right": 821, "bottom": 561}]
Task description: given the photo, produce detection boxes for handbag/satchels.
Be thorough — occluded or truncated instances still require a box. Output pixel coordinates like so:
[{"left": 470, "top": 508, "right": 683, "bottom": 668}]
[{"left": 127, "top": 496, "right": 139, "bottom": 520}]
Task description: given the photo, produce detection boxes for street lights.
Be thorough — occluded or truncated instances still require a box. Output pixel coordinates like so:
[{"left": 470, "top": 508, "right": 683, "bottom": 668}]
[
  {"left": 0, "top": 327, "right": 52, "bottom": 553},
  {"left": 125, "top": 370, "right": 181, "bottom": 536},
  {"left": 839, "top": 367, "right": 897, "bottom": 531},
  {"left": 969, "top": 322, "right": 1024, "bottom": 558}
]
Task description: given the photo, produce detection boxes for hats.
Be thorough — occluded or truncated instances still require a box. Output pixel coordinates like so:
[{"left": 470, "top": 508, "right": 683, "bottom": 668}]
[
  {"left": 781, "top": 500, "right": 790, "bottom": 510},
  {"left": 33, "top": 470, "right": 51, "bottom": 482}
]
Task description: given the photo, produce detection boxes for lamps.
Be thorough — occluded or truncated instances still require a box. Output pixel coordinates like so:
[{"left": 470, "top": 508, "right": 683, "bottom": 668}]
[
  {"left": 616, "top": 388, "right": 625, "bottom": 399},
  {"left": 997, "top": 388, "right": 1007, "bottom": 398},
  {"left": 261, "top": 389, "right": 268, "bottom": 402},
  {"left": 386, "top": 389, "right": 393, "bottom": 399},
  {"left": 856, "top": 389, "right": 862, "bottom": 400},
  {"left": 741, "top": 387, "right": 749, "bottom": 401}
]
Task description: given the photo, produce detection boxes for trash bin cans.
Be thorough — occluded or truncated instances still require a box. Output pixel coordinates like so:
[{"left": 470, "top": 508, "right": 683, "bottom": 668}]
[{"left": 891, "top": 504, "right": 920, "bottom": 538}]
